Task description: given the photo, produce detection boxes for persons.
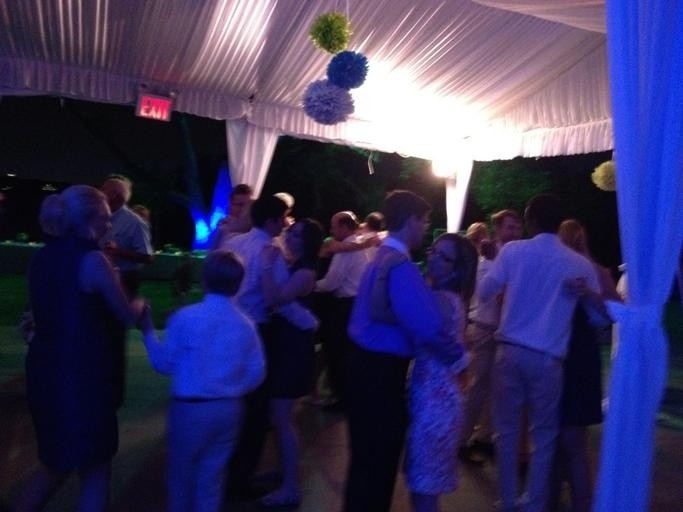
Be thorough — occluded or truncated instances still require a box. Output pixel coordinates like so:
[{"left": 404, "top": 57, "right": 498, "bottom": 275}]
[
  {"left": 138, "top": 247, "right": 266, "bottom": 510},
  {"left": 9, "top": 184, "right": 151, "bottom": 510},
  {"left": 316, "top": 212, "right": 387, "bottom": 411},
  {"left": 341, "top": 191, "right": 479, "bottom": 512},
  {"left": 211, "top": 183, "right": 322, "bottom": 511},
  {"left": 460, "top": 211, "right": 524, "bottom": 463},
  {"left": 476, "top": 195, "right": 622, "bottom": 512},
  {"left": 131, "top": 205, "right": 148, "bottom": 219},
  {"left": 97, "top": 173, "right": 151, "bottom": 410}
]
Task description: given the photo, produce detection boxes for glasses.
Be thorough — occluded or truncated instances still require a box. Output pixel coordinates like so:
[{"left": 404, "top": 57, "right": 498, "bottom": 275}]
[{"left": 427, "top": 247, "right": 453, "bottom": 262}]
